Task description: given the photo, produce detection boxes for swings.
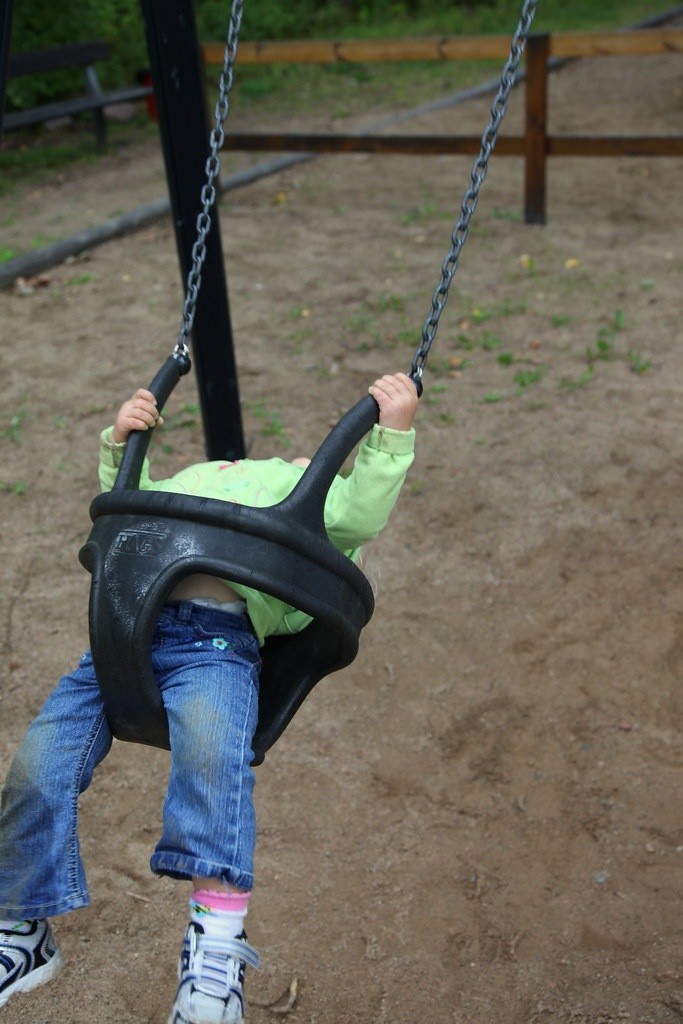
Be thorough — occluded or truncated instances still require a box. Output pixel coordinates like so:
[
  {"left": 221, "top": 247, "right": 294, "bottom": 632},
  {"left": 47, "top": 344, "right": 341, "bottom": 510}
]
[{"left": 73, "top": 0, "right": 542, "bottom": 767}]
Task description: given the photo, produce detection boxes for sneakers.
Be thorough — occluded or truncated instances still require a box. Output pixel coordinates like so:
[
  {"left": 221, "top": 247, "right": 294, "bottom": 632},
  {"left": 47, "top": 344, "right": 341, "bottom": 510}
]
[
  {"left": 1, "top": 918, "right": 62, "bottom": 1008},
  {"left": 166, "top": 929, "right": 261, "bottom": 1022}
]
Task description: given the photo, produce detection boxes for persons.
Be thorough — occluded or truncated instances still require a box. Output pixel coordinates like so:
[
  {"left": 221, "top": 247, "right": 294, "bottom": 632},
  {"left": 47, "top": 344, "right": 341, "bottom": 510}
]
[{"left": 1, "top": 371, "right": 416, "bottom": 1022}]
